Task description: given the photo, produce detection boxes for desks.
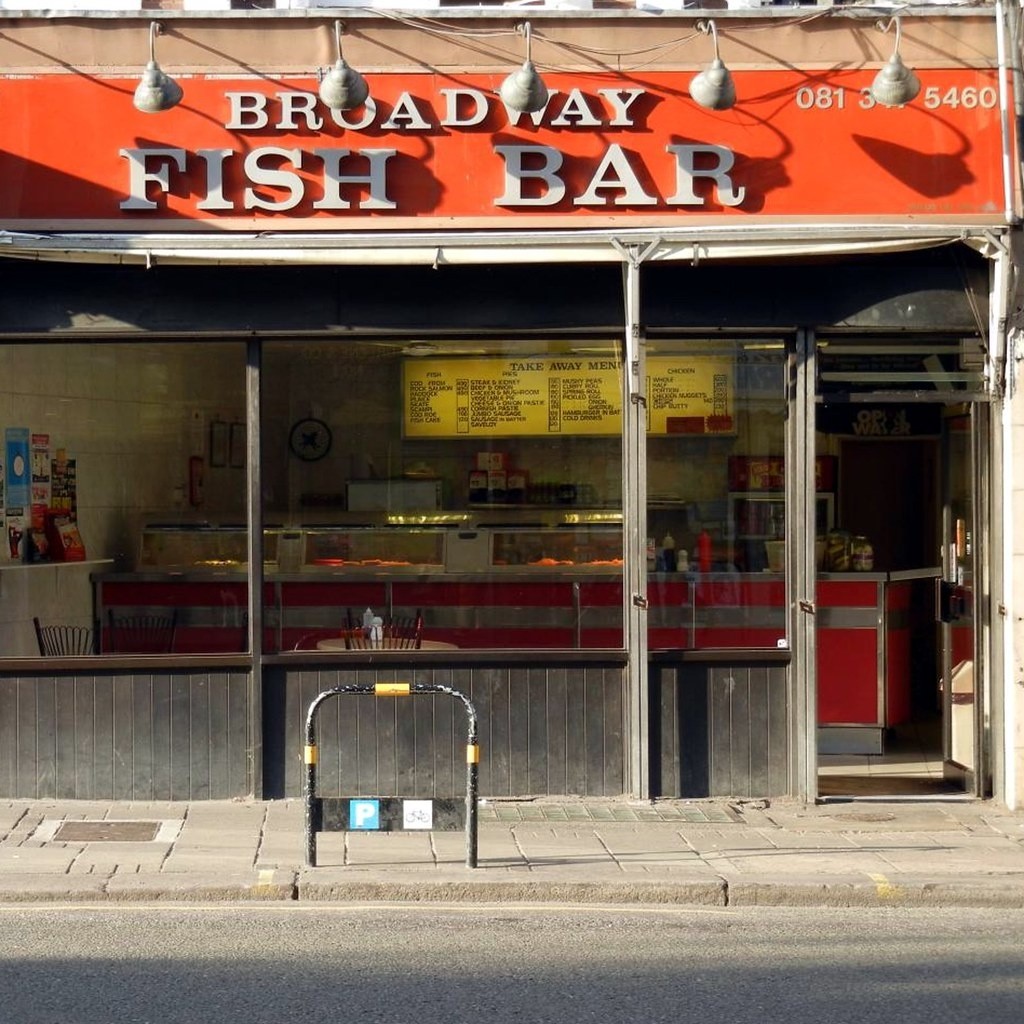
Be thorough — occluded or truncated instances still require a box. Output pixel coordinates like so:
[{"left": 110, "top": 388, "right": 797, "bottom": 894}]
[{"left": 315, "top": 636, "right": 460, "bottom": 650}]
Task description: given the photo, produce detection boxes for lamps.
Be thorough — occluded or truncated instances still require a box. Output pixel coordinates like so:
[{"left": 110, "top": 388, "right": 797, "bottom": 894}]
[
  {"left": 319, "top": 17, "right": 370, "bottom": 111},
  {"left": 871, "top": 16, "right": 921, "bottom": 108},
  {"left": 498, "top": 19, "right": 549, "bottom": 115},
  {"left": 132, "top": 19, "right": 184, "bottom": 113},
  {"left": 689, "top": 18, "right": 737, "bottom": 110}
]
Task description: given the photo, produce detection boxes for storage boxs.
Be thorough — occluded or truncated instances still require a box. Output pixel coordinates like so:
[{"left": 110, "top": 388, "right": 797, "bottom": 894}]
[{"left": 727, "top": 454, "right": 837, "bottom": 491}]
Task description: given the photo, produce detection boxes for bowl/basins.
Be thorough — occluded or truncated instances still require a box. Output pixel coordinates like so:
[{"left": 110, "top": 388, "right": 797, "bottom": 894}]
[{"left": 764, "top": 541, "right": 826, "bottom": 572}]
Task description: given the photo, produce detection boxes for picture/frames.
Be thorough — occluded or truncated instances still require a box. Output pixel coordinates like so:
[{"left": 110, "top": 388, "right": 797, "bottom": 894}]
[
  {"left": 230, "top": 423, "right": 248, "bottom": 466},
  {"left": 211, "top": 420, "right": 226, "bottom": 465}
]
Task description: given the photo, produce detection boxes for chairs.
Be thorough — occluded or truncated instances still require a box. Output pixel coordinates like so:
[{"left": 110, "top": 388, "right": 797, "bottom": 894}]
[
  {"left": 343, "top": 608, "right": 429, "bottom": 657},
  {"left": 108, "top": 606, "right": 180, "bottom": 657},
  {"left": 34, "top": 615, "right": 103, "bottom": 657}
]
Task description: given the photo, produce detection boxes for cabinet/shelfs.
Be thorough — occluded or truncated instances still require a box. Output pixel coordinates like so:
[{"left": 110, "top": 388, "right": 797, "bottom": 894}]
[{"left": 728, "top": 492, "right": 834, "bottom": 570}]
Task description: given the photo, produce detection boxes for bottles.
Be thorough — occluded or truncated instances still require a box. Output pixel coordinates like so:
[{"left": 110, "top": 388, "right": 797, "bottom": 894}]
[
  {"left": 696, "top": 529, "right": 713, "bottom": 571},
  {"left": 363, "top": 607, "right": 374, "bottom": 627},
  {"left": 370, "top": 616, "right": 382, "bottom": 641},
  {"left": 826, "top": 527, "right": 874, "bottom": 571},
  {"left": 678, "top": 550, "right": 688, "bottom": 572},
  {"left": 662, "top": 532, "right": 675, "bottom": 572}
]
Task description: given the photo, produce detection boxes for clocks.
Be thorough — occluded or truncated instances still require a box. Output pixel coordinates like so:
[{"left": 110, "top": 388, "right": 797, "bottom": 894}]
[{"left": 291, "top": 416, "right": 333, "bottom": 459}]
